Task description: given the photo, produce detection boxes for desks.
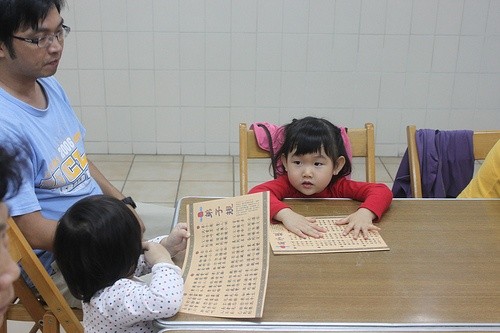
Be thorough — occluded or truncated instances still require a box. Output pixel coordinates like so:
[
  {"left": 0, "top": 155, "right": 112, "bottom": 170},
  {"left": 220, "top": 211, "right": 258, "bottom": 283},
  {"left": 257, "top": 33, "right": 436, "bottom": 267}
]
[{"left": 152, "top": 195, "right": 500, "bottom": 333}]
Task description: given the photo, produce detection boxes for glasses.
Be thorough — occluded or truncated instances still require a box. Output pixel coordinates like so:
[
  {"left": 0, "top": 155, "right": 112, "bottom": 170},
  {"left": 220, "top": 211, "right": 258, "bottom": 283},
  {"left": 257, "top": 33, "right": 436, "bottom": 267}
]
[{"left": 11, "top": 25, "right": 70, "bottom": 48}]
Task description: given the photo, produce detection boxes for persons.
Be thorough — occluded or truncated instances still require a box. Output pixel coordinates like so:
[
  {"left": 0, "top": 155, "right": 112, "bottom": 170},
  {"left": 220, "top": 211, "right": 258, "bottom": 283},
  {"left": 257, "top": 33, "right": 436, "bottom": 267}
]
[
  {"left": 248, "top": 116, "right": 392, "bottom": 240},
  {"left": 454, "top": 136, "right": 500, "bottom": 198},
  {"left": 0, "top": 138, "right": 29, "bottom": 331},
  {"left": 0, "top": 0, "right": 146, "bottom": 309},
  {"left": 53, "top": 195, "right": 191, "bottom": 333}
]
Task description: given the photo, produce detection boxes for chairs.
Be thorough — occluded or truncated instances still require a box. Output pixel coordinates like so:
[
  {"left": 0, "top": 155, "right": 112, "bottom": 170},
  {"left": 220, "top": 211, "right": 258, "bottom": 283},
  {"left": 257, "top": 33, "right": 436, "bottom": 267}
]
[
  {"left": 239, "top": 123, "right": 375, "bottom": 196},
  {"left": 406, "top": 124, "right": 500, "bottom": 199},
  {"left": 0, "top": 216, "right": 84, "bottom": 333}
]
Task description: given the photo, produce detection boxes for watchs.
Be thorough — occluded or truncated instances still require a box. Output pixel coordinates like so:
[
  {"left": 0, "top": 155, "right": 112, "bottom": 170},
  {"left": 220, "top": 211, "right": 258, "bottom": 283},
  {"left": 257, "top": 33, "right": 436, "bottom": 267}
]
[{"left": 121, "top": 196, "right": 136, "bottom": 209}]
